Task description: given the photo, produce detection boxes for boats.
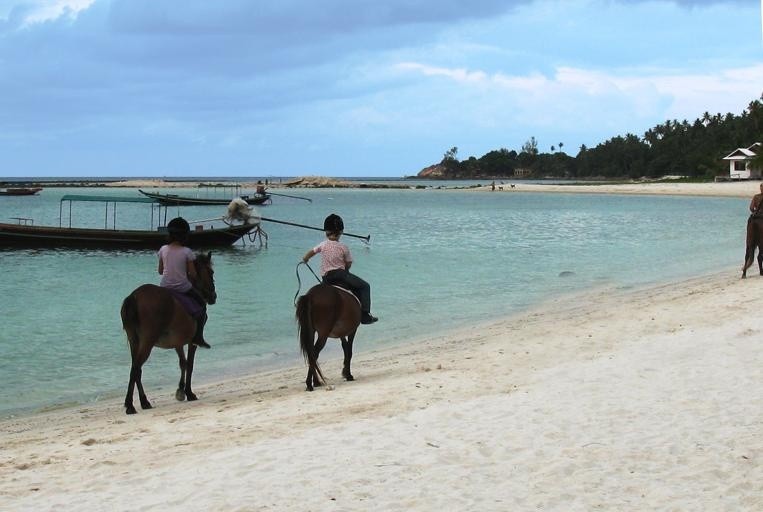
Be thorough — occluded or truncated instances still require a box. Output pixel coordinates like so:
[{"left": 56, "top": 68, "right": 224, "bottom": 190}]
[{"left": 0, "top": 182, "right": 273, "bottom": 255}]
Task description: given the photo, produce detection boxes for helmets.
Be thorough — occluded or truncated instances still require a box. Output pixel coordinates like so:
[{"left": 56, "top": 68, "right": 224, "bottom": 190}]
[
  {"left": 167, "top": 218, "right": 190, "bottom": 233},
  {"left": 324, "top": 214, "right": 343, "bottom": 231}
]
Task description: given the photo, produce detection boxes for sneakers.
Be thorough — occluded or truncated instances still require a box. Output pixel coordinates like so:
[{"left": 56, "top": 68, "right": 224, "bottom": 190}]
[
  {"left": 361, "top": 314, "right": 378, "bottom": 324},
  {"left": 192, "top": 335, "right": 210, "bottom": 348}
]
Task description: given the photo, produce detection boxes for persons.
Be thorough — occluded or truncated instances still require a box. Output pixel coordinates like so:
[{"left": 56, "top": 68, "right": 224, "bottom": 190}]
[
  {"left": 156, "top": 216, "right": 213, "bottom": 351},
  {"left": 304, "top": 212, "right": 378, "bottom": 327},
  {"left": 749, "top": 183, "right": 763, "bottom": 216}
]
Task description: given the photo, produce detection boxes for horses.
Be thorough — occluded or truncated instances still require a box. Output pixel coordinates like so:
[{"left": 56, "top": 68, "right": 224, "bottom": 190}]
[
  {"left": 741, "top": 212, "right": 763, "bottom": 280},
  {"left": 120, "top": 248, "right": 220, "bottom": 416},
  {"left": 295, "top": 280, "right": 364, "bottom": 391}
]
